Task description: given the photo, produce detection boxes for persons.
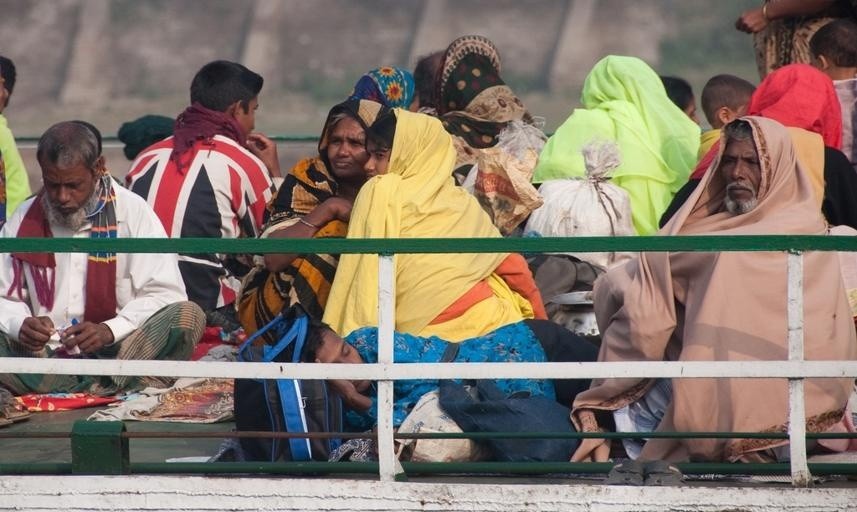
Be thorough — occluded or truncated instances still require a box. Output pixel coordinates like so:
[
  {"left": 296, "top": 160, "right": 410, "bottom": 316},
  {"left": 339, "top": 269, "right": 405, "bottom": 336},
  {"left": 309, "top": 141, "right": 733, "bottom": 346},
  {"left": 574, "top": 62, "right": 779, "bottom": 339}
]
[
  {"left": 124, "top": 60, "right": 284, "bottom": 330},
  {"left": 809, "top": 21, "right": 856, "bottom": 162},
  {"left": 570, "top": 120, "right": 857, "bottom": 466},
  {"left": 658, "top": 76, "right": 700, "bottom": 127},
  {"left": 410, "top": 35, "right": 549, "bottom": 236},
  {"left": 322, "top": 108, "right": 549, "bottom": 343},
  {"left": 701, "top": 74, "right": 757, "bottom": 130},
  {"left": 0, "top": 120, "right": 206, "bottom": 425},
  {"left": 533, "top": 54, "right": 701, "bottom": 236},
  {"left": 239, "top": 99, "right": 387, "bottom": 346},
  {"left": 734, "top": 1, "right": 857, "bottom": 82},
  {"left": 273, "top": 318, "right": 614, "bottom": 440},
  {"left": 0, "top": 54, "right": 33, "bottom": 231},
  {"left": 659, "top": 63, "right": 856, "bottom": 231},
  {"left": 349, "top": 64, "right": 414, "bottom": 110}
]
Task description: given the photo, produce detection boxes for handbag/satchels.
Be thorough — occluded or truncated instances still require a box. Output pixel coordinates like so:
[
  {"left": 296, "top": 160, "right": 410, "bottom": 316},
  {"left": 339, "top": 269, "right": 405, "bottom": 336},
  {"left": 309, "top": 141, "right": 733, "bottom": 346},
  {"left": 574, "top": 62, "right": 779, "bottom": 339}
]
[{"left": 236, "top": 303, "right": 344, "bottom": 463}]
